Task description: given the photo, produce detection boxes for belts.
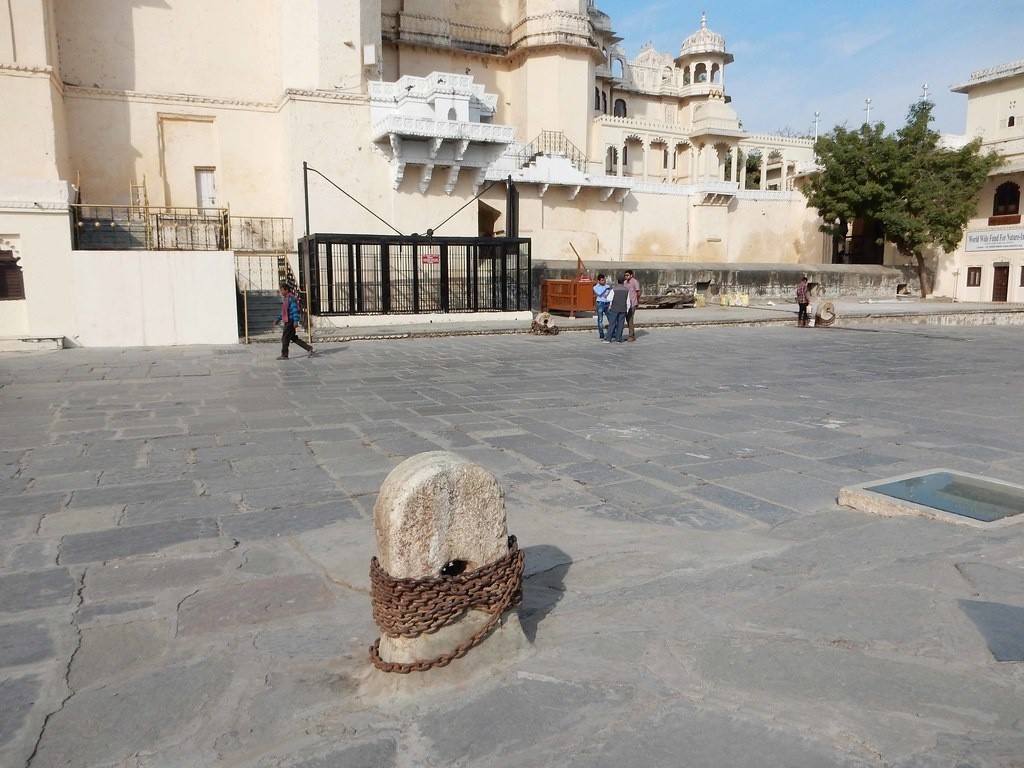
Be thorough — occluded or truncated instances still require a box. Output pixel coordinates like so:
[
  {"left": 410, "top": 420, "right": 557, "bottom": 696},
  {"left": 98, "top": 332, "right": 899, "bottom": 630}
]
[{"left": 597, "top": 301, "right": 610, "bottom": 305}]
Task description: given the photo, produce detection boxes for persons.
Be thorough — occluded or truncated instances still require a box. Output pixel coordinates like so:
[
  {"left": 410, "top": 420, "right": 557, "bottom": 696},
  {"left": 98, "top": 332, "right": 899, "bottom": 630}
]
[
  {"left": 603, "top": 276, "right": 630, "bottom": 344},
  {"left": 796, "top": 278, "right": 812, "bottom": 328},
  {"left": 593, "top": 274, "right": 616, "bottom": 340},
  {"left": 624, "top": 270, "right": 641, "bottom": 342},
  {"left": 273, "top": 283, "right": 313, "bottom": 360}
]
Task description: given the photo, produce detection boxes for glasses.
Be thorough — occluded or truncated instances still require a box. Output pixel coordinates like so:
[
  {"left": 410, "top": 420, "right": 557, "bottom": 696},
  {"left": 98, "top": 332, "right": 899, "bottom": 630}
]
[{"left": 624, "top": 275, "right": 629, "bottom": 277}]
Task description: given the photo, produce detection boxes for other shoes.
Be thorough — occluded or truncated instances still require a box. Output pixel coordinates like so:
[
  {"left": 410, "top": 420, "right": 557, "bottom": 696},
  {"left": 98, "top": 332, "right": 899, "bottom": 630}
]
[{"left": 624, "top": 336, "right": 635, "bottom": 342}]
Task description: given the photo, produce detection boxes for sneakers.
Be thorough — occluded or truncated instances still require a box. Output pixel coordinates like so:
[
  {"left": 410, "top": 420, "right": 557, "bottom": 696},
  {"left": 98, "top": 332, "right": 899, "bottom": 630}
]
[
  {"left": 600, "top": 338, "right": 604, "bottom": 341},
  {"left": 276, "top": 356, "right": 288, "bottom": 360},
  {"left": 603, "top": 340, "right": 610, "bottom": 344},
  {"left": 616, "top": 338, "right": 622, "bottom": 344},
  {"left": 307, "top": 348, "right": 313, "bottom": 358}
]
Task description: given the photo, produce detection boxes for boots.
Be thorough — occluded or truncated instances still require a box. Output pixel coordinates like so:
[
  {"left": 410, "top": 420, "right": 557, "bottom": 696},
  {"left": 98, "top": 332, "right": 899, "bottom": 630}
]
[
  {"left": 805, "top": 320, "right": 811, "bottom": 328},
  {"left": 798, "top": 319, "right": 805, "bottom": 327}
]
